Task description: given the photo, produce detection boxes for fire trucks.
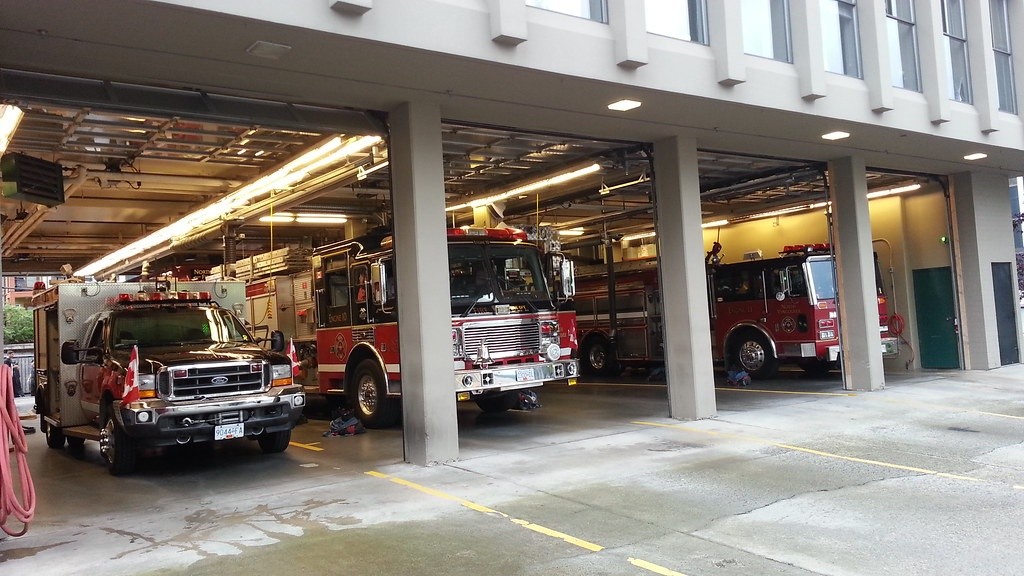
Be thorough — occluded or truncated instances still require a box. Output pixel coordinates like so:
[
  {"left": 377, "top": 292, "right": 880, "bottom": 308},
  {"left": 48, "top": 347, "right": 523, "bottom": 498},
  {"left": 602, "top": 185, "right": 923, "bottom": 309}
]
[
  {"left": 526, "top": 242, "right": 901, "bottom": 379},
  {"left": 27, "top": 265, "right": 307, "bottom": 476},
  {"left": 203, "top": 221, "right": 585, "bottom": 429}
]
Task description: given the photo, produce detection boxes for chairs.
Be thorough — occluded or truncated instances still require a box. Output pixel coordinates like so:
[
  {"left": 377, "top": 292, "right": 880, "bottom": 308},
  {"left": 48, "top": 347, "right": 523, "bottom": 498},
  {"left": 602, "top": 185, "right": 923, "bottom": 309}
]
[
  {"left": 182, "top": 328, "right": 206, "bottom": 341},
  {"left": 116, "top": 331, "right": 134, "bottom": 344}
]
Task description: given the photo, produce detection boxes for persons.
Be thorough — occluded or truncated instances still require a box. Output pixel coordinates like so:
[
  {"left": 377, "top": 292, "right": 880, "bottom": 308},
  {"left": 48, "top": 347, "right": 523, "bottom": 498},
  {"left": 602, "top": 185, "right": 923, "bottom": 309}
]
[
  {"left": 358, "top": 271, "right": 366, "bottom": 302},
  {"left": 4, "top": 351, "right": 35, "bottom": 397}
]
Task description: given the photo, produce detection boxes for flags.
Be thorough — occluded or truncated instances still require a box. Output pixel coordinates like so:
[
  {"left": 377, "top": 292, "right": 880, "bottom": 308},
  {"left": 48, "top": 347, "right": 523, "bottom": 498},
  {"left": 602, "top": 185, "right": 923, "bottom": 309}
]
[
  {"left": 119, "top": 345, "right": 139, "bottom": 409},
  {"left": 286, "top": 340, "right": 301, "bottom": 377}
]
